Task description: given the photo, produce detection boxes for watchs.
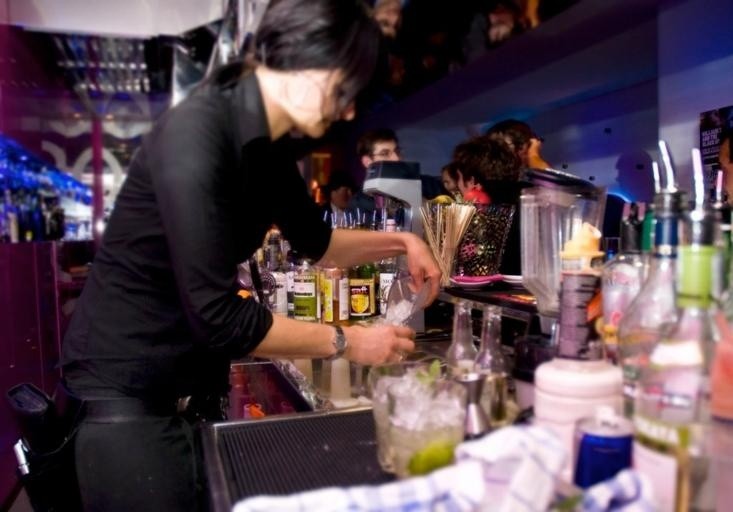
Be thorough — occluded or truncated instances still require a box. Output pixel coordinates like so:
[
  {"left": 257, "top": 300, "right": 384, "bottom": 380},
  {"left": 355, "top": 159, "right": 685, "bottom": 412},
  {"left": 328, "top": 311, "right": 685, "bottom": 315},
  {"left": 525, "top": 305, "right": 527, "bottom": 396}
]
[{"left": 324, "top": 323, "right": 348, "bottom": 365}]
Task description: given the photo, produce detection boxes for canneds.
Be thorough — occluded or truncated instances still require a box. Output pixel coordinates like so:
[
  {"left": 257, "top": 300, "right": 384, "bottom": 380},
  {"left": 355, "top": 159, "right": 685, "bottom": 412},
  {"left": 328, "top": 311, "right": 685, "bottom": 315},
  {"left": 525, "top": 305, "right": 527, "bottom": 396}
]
[
  {"left": 571, "top": 415, "right": 634, "bottom": 490},
  {"left": 558, "top": 269, "right": 601, "bottom": 362}
]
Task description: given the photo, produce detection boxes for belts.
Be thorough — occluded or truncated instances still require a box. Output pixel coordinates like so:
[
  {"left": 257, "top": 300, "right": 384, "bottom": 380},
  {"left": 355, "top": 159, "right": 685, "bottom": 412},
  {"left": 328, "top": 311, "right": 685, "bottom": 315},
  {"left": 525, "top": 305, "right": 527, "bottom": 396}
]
[{"left": 78, "top": 391, "right": 197, "bottom": 425}]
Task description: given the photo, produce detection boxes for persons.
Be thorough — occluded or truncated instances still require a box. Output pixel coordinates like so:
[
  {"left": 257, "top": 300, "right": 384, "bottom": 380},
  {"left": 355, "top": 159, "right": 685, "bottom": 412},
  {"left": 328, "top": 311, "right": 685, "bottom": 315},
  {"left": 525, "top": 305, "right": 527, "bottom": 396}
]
[{"left": 52, "top": 0, "right": 445, "bottom": 511}]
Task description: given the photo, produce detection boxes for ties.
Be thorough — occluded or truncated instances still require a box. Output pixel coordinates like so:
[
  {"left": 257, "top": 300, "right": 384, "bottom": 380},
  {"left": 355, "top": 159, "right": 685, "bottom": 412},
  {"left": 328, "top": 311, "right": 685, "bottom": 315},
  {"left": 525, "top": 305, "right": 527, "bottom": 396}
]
[{"left": 368, "top": 150, "right": 402, "bottom": 156}]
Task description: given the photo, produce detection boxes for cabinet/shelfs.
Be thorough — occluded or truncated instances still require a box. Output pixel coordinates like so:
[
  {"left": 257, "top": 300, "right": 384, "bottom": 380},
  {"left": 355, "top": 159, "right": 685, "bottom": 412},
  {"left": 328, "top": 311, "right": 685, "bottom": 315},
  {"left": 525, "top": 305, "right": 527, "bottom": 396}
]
[{"left": 0, "top": 243, "right": 94, "bottom": 508}]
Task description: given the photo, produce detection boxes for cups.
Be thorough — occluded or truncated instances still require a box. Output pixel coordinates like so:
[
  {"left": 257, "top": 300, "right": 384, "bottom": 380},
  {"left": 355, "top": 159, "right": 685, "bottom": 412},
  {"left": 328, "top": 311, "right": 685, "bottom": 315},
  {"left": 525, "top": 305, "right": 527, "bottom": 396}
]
[
  {"left": 227, "top": 359, "right": 298, "bottom": 419},
  {"left": 372, "top": 318, "right": 412, "bottom": 364},
  {"left": 369, "top": 361, "right": 468, "bottom": 480},
  {"left": 430, "top": 247, "right": 461, "bottom": 290}
]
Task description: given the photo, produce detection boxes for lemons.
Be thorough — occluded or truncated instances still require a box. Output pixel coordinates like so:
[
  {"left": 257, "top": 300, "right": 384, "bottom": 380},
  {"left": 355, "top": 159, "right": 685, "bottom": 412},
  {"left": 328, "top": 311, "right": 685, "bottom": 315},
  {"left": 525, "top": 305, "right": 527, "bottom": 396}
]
[{"left": 408, "top": 440, "right": 455, "bottom": 474}]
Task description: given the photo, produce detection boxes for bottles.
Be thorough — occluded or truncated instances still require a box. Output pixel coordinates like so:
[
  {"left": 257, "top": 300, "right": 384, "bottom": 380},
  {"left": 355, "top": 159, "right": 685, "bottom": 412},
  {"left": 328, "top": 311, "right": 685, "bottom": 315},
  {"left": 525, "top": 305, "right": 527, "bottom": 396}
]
[
  {"left": 0, "top": 135, "right": 97, "bottom": 244},
  {"left": 450, "top": 300, "right": 516, "bottom": 430},
  {"left": 607, "top": 189, "right": 733, "bottom": 512},
  {"left": 262, "top": 218, "right": 402, "bottom": 327}
]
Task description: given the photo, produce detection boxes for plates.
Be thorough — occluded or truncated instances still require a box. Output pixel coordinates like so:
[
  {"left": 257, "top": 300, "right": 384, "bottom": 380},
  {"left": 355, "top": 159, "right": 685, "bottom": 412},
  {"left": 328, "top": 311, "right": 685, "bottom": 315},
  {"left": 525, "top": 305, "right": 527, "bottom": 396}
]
[
  {"left": 501, "top": 273, "right": 529, "bottom": 290},
  {"left": 448, "top": 277, "right": 491, "bottom": 290}
]
